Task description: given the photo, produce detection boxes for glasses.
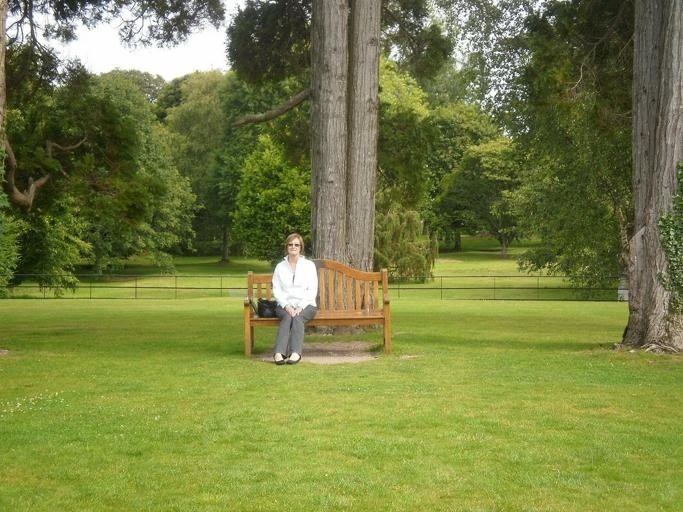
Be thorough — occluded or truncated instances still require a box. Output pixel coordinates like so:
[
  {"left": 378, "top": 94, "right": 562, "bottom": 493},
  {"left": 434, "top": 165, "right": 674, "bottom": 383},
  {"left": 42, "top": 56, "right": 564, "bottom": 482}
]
[{"left": 287, "top": 243, "right": 302, "bottom": 247}]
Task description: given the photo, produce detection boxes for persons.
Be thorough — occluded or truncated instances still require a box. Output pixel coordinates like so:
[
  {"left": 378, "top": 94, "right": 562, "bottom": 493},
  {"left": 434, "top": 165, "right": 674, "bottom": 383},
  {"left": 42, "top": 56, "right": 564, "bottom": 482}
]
[{"left": 272, "top": 234, "right": 319, "bottom": 365}]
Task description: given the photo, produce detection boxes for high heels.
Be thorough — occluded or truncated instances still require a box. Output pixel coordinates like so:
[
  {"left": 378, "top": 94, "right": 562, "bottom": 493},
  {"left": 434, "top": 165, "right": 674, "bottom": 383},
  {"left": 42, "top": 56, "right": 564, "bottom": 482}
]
[{"left": 273, "top": 355, "right": 301, "bottom": 365}]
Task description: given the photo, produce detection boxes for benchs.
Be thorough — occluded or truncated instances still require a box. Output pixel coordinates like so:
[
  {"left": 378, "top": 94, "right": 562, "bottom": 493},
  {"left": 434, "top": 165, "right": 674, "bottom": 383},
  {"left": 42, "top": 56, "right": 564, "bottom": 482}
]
[{"left": 242, "top": 257, "right": 394, "bottom": 356}]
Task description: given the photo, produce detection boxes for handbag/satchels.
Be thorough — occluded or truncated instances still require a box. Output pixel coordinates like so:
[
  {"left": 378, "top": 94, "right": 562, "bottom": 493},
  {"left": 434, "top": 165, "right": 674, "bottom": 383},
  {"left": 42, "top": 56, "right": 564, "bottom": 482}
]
[{"left": 257, "top": 298, "right": 277, "bottom": 318}]
